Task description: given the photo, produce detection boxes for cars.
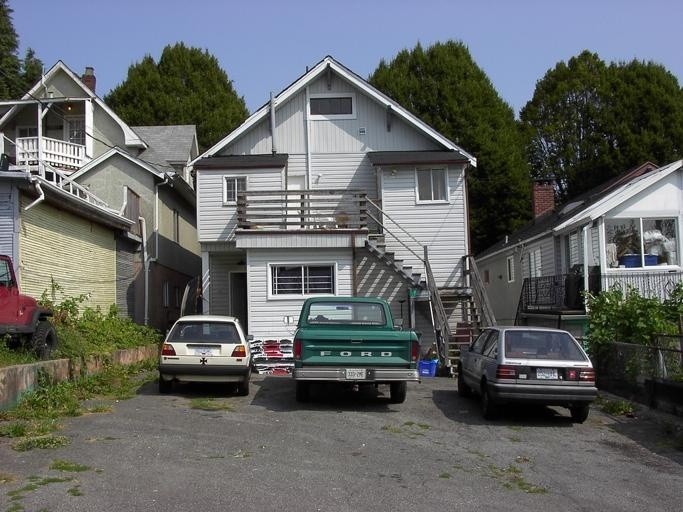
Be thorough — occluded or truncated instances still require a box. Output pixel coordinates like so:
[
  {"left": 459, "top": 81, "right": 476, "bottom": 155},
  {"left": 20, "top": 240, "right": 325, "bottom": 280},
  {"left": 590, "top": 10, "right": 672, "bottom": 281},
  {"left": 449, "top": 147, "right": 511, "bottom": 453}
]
[
  {"left": 156, "top": 313, "right": 255, "bottom": 396},
  {"left": 457, "top": 325, "right": 599, "bottom": 425}
]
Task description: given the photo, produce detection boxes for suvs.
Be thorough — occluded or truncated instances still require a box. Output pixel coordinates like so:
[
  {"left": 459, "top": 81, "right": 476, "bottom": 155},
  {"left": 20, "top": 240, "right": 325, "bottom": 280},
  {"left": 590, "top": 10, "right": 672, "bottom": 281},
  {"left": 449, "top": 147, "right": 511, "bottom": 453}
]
[{"left": 0, "top": 251, "right": 58, "bottom": 359}]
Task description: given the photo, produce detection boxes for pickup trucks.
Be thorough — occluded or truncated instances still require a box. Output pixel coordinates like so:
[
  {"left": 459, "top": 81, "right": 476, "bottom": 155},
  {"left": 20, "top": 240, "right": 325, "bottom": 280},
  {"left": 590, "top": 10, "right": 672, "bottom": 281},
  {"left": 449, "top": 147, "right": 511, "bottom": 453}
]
[{"left": 288, "top": 293, "right": 423, "bottom": 407}]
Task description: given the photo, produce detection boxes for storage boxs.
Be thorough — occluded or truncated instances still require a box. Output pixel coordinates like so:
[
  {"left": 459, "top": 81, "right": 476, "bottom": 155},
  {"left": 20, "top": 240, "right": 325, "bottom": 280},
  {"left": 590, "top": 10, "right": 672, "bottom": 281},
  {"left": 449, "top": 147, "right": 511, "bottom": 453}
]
[
  {"left": 622, "top": 252, "right": 659, "bottom": 269},
  {"left": 417, "top": 359, "right": 438, "bottom": 378}
]
